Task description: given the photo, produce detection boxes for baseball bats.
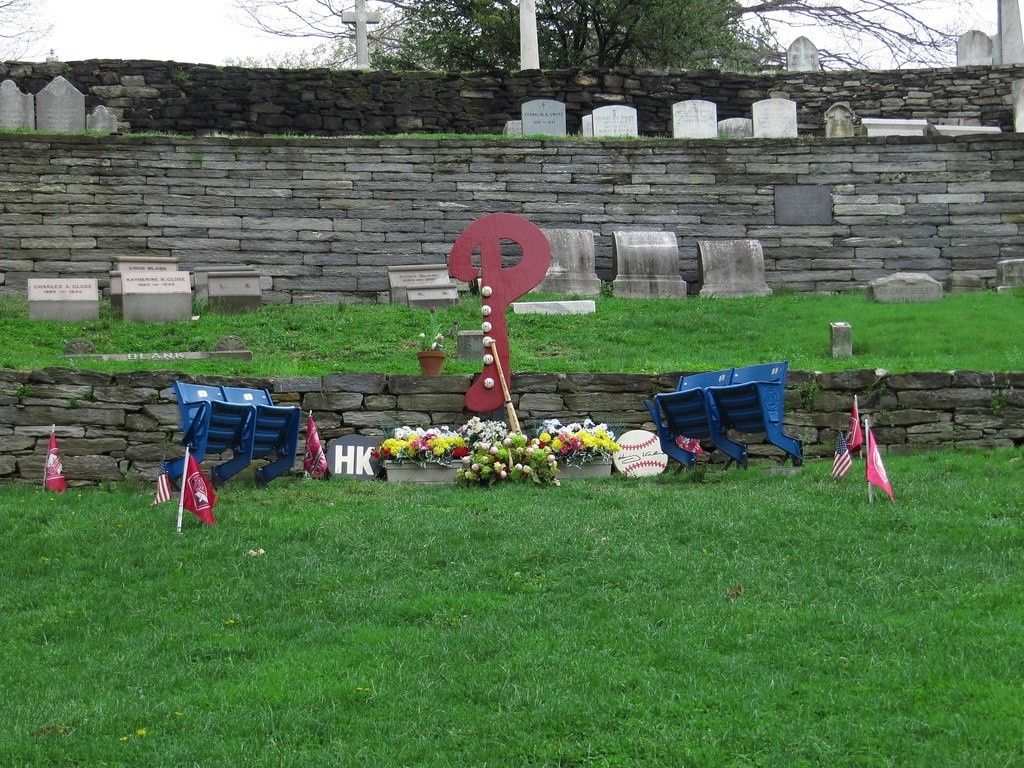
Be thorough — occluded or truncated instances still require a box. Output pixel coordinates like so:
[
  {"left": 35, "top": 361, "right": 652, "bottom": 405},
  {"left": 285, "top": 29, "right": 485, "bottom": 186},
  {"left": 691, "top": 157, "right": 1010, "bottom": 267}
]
[{"left": 488, "top": 339, "right": 522, "bottom": 433}]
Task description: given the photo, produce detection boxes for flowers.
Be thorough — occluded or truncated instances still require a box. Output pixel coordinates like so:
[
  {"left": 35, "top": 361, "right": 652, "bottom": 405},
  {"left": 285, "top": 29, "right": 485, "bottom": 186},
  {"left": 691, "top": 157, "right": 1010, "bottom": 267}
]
[
  {"left": 457, "top": 437, "right": 560, "bottom": 487},
  {"left": 417, "top": 306, "right": 444, "bottom": 351},
  {"left": 369, "top": 416, "right": 621, "bottom": 468}
]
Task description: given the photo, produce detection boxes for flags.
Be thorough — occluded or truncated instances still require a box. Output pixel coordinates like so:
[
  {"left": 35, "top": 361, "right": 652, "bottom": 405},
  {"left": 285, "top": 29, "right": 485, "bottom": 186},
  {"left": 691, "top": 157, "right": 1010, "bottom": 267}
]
[
  {"left": 832, "top": 394, "right": 895, "bottom": 504},
  {"left": 149, "top": 458, "right": 174, "bottom": 506},
  {"left": 45, "top": 429, "right": 67, "bottom": 495},
  {"left": 303, "top": 410, "right": 327, "bottom": 479},
  {"left": 180, "top": 454, "right": 218, "bottom": 526}
]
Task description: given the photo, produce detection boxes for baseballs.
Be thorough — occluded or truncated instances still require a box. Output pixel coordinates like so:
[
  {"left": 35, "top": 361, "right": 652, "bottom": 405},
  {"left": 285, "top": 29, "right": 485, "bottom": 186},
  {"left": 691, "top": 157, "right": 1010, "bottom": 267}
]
[{"left": 612, "top": 429, "right": 668, "bottom": 476}]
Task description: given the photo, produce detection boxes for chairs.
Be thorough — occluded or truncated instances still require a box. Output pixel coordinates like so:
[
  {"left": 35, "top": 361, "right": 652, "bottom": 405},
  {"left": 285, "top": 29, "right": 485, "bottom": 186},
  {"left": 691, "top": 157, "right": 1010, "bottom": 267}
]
[
  {"left": 165, "top": 380, "right": 301, "bottom": 488},
  {"left": 645, "top": 360, "right": 803, "bottom": 477}
]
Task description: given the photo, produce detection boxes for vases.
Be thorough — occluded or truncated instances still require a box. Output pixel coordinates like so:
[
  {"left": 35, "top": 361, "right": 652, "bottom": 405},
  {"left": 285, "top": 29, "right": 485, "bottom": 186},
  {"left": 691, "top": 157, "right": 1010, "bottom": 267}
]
[
  {"left": 383, "top": 456, "right": 612, "bottom": 482},
  {"left": 418, "top": 350, "right": 446, "bottom": 375}
]
